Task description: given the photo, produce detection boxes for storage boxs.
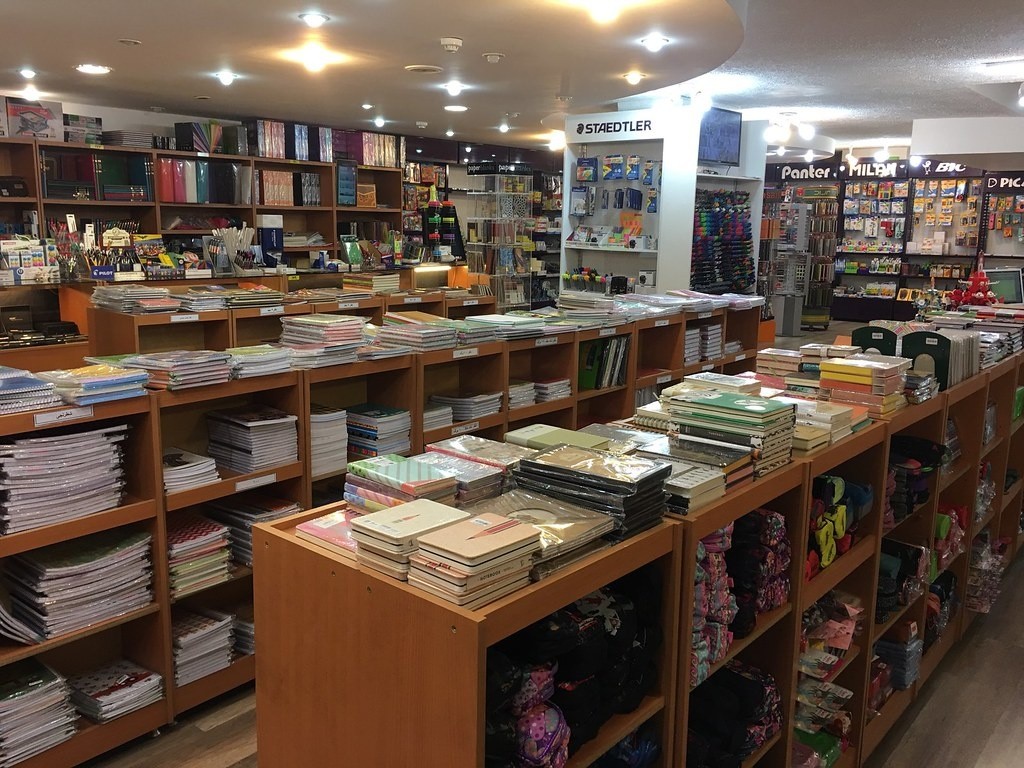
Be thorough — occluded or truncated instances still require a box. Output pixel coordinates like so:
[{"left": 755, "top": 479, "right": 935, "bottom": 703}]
[
  {"left": 261, "top": 170, "right": 269, "bottom": 205},
  {"left": 195, "top": 161, "right": 208, "bottom": 203},
  {"left": 307, "top": 126, "right": 327, "bottom": 162},
  {"left": 158, "top": 159, "right": 174, "bottom": 203},
  {"left": 268, "top": 171, "right": 275, "bottom": 207},
  {"left": 285, "top": 124, "right": 302, "bottom": 160},
  {"left": 278, "top": 172, "right": 285, "bottom": 206},
  {"left": 325, "top": 128, "right": 333, "bottom": 163},
  {"left": 277, "top": 123, "right": 285, "bottom": 159},
  {"left": 253, "top": 170, "right": 260, "bottom": 203},
  {"left": 300, "top": 126, "right": 309, "bottom": 161},
  {"left": 283, "top": 172, "right": 289, "bottom": 206},
  {"left": 185, "top": 161, "right": 198, "bottom": 204},
  {"left": 210, "top": 163, "right": 242, "bottom": 206},
  {"left": 172, "top": 159, "right": 186, "bottom": 202},
  {"left": 274, "top": 171, "right": 280, "bottom": 205},
  {"left": 241, "top": 120, "right": 266, "bottom": 158},
  {"left": 262, "top": 121, "right": 273, "bottom": 157},
  {"left": 222, "top": 126, "right": 249, "bottom": 155},
  {"left": 270, "top": 122, "right": 279, "bottom": 158}
]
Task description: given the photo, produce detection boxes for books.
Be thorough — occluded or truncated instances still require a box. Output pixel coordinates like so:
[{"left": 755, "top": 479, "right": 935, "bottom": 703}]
[
  {"left": 157, "top": 119, "right": 406, "bottom": 207},
  {"left": 159, "top": 445, "right": 223, "bottom": 494},
  {"left": 119, "top": 347, "right": 231, "bottom": 391},
  {"left": 309, "top": 374, "right": 573, "bottom": 480},
  {"left": 89, "top": 270, "right": 400, "bottom": 317},
  {"left": 359, "top": 289, "right": 768, "bottom": 361},
  {"left": 868, "top": 304, "right": 980, "bottom": 388},
  {"left": 63, "top": 113, "right": 153, "bottom": 148},
  {"left": 972, "top": 303, "right": 1024, "bottom": 370},
  {"left": 279, "top": 312, "right": 364, "bottom": 370},
  {"left": 167, "top": 489, "right": 313, "bottom": 690},
  {"left": 39, "top": 150, "right": 156, "bottom": 203},
  {"left": 224, "top": 343, "right": 296, "bottom": 380},
  {"left": 577, "top": 336, "right": 631, "bottom": 389},
  {"left": 203, "top": 400, "right": 299, "bottom": 473},
  {"left": 681, "top": 322, "right": 723, "bottom": 366},
  {"left": 343, "top": 341, "right": 937, "bottom": 613},
  {"left": 162, "top": 215, "right": 403, "bottom": 268},
  {"left": 0, "top": 362, "right": 166, "bottom": 768}
]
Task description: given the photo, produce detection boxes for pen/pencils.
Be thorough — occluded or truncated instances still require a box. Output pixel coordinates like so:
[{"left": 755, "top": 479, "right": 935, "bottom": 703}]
[
  {"left": 54, "top": 242, "right": 91, "bottom": 278},
  {"left": 88, "top": 248, "right": 137, "bottom": 271},
  {"left": 46, "top": 216, "right": 67, "bottom": 244},
  {"left": 92, "top": 217, "right": 141, "bottom": 249}
]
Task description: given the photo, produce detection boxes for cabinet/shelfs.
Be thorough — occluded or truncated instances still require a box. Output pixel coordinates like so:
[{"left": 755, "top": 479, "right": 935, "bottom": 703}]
[{"left": 0, "top": 113, "right": 1024, "bottom": 768}]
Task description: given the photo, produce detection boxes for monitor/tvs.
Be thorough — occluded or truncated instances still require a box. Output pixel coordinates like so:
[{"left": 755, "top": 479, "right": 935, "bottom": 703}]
[
  {"left": 982, "top": 269, "right": 1024, "bottom": 306},
  {"left": 698, "top": 107, "right": 742, "bottom": 167}
]
